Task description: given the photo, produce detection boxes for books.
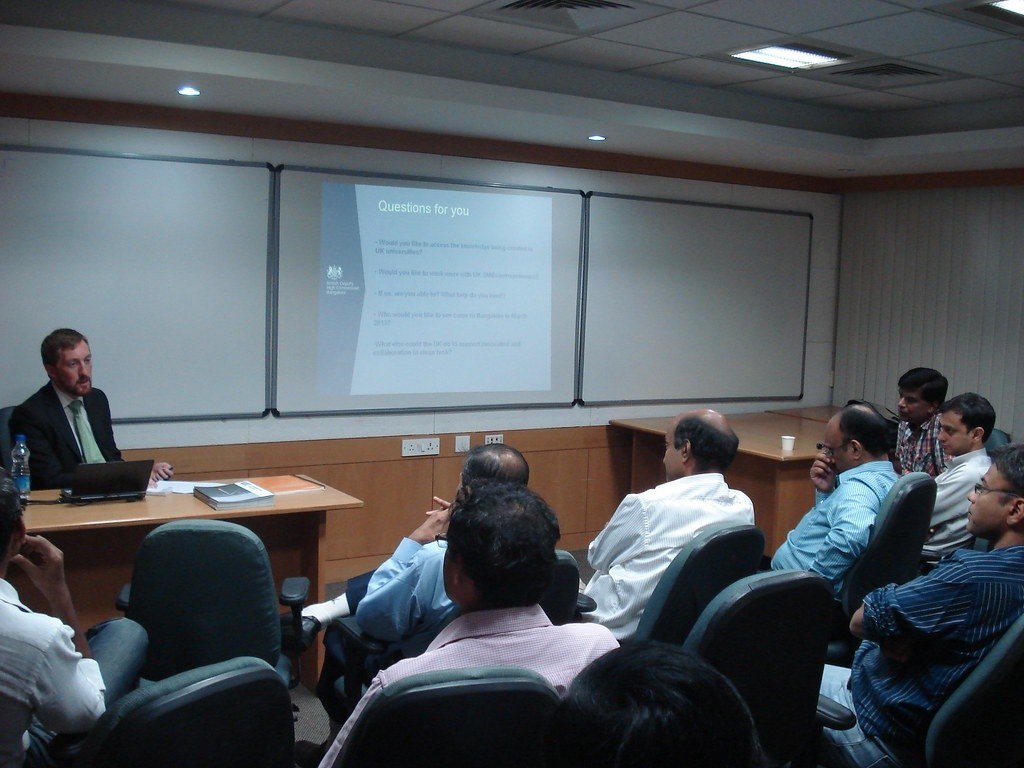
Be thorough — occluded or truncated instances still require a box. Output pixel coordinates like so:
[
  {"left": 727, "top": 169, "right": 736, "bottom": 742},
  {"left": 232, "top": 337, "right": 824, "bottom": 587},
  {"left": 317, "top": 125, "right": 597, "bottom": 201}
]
[{"left": 193, "top": 481, "right": 274, "bottom": 511}]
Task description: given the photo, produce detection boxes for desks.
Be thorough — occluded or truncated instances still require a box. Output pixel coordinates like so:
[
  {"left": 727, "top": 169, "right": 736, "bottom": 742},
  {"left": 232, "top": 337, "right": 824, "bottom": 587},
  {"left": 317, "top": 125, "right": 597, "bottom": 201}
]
[
  {"left": 7, "top": 473, "right": 365, "bottom": 697},
  {"left": 611, "top": 406, "right": 845, "bottom": 558}
]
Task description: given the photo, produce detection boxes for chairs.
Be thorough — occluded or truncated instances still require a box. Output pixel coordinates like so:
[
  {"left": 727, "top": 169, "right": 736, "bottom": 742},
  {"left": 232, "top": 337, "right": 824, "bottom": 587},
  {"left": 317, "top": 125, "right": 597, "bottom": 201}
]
[
  {"left": 80, "top": 497, "right": 858, "bottom": 768},
  {"left": 827, "top": 430, "right": 1024, "bottom": 768},
  {"left": 0, "top": 406, "right": 17, "bottom": 472}
]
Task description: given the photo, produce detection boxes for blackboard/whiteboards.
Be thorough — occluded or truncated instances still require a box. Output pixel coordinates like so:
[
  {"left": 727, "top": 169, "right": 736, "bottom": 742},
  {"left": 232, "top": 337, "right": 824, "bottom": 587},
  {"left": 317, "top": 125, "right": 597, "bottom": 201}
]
[
  {"left": 578, "top": 190, "right": 813, "bottom": 405},
  {"left": 0, "top": 143, "right": 271, "bottom": 425},
  {"left": 273, "top": 165, "right": 584, "bottom": 418}
]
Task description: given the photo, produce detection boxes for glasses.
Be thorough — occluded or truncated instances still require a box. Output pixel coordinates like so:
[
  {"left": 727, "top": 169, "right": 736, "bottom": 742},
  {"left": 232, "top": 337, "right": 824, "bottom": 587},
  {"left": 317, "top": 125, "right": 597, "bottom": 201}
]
[
  {"left": 435, "top": 532, "right": 453, "bottom": 548},
  {"left": 975, "top": 483, "right": 1024, "bottom": 498},
  {"left": 823, "top": 439, "right": 859, "bottom": 459}
]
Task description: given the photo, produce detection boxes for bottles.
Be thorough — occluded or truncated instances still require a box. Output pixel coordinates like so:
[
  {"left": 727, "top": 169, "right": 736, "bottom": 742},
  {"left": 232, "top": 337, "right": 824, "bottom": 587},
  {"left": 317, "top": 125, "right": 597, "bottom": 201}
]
[{"left": 11, "top": 436, "right": 30, "bottom": 496}]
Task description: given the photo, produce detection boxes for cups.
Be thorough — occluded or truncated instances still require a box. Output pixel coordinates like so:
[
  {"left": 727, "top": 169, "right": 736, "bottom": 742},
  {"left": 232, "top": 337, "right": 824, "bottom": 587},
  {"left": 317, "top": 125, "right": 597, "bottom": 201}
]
[{"left": 782, "top": 436, "right": 795, "bottom": 451}]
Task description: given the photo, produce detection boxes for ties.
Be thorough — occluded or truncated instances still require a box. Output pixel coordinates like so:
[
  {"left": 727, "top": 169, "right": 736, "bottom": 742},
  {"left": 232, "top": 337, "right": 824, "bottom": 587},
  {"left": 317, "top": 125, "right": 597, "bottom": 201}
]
[{"left": 68, "top": 399, "right": 106, "bottom": 464}]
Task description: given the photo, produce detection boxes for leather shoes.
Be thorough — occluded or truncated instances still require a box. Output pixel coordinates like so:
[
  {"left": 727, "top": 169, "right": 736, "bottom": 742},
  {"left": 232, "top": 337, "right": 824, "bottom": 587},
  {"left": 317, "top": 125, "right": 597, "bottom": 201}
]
[{"left": 279, "top": 610, "right": 321, "bottom": 652}]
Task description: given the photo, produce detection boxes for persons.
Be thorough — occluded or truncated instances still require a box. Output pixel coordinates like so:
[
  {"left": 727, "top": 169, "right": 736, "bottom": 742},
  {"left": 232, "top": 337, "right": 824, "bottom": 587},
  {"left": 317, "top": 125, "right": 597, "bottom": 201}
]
[
  {"left": 760, "top": 404, "right": 901, "bottom": 642},
  {"left": 579, "top": 409, "right": 755, "bottom": 640},
  {"left": 543, "top": 639, "right": 767, "bottom": 768},
  {"left": 924, "top": 393, "right": 996, "bottom": 544},
  {"left": 792, "top": 442, "right": 1024, "bottom": 768},
  {"left": 316, "top": 479, "right": 621, "bottom": 768},
  {"left": 9, "top": 329, "right": 173, "bottom": 491},
  {"left": 895, "top": 368, "right": 956, "bottom": 476},
  {"left": 279, "top": 439, "right": 529, "bottom": 768},
  {"left": 0, "top": 467, "right": 148, "bottom": 768}
]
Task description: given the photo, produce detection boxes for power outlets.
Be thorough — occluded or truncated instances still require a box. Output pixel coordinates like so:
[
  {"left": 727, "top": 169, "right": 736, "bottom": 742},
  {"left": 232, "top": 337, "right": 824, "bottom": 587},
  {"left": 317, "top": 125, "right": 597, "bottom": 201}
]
[{"left": 402, "top": 438, "right": 440, "bottom": 457}]
